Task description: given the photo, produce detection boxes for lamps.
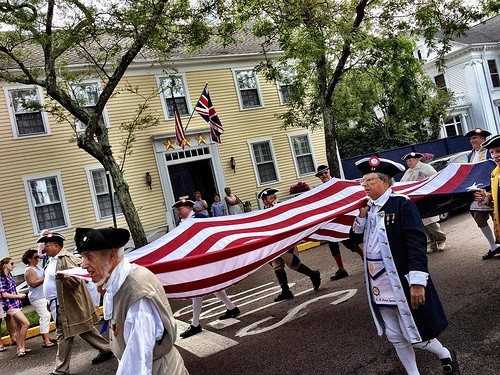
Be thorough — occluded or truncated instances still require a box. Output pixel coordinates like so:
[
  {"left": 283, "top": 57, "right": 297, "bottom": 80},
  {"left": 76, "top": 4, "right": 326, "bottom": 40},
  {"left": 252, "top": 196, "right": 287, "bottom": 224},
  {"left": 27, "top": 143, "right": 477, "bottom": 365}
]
[
  {"left": 145, "top": 171, "right": 152, "bottom": 189},
  {"left": 230, "top": 156, "right": 236, "bottom": 173}
]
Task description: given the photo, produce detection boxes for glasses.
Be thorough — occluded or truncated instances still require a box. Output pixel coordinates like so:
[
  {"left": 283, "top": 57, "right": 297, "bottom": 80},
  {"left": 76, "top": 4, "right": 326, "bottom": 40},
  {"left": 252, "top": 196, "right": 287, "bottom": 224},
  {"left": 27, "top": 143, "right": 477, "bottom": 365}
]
[
  {"left": 34, "top": 256, "right": 39, "bottom": 259},
  {"left": 11, "top": 262, "right": 14, "bottom": 266},
  {"left": 318, "top": 172, "right": 328, "bottom": 176},
  {"left": 360, "top": 177, "right": 383, "bottom": 185}
]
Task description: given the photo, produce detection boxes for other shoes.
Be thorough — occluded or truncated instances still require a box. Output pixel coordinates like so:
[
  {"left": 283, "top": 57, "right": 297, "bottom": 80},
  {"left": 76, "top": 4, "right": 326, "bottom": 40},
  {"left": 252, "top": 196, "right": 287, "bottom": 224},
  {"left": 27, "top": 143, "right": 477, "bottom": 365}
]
[
  {"left": 92, "top": 350, "right": 115, "bottom": 365},
  {"left": 180, "top": 324, "right": 202, "bottom": 337},
  {"left": 330, "top": 269, "right": 348, "bottom": 279},
  {"left": 438, "top": 243, "right": 445, "bottom": 250},
  {"left": 427, "top": 246, "right": 437, "bottom": 253},
  {"left": 273, "top": 291, "right": 294, "bottom": 302},
  {"left": 219, "top": 306, "right": 240, "bottom": 320},
  {"left": 47, "top": 372, "right": 58, "bottom": 375},
  {"left": 310, "top": 268, "right": 321, "bottom": 292}
]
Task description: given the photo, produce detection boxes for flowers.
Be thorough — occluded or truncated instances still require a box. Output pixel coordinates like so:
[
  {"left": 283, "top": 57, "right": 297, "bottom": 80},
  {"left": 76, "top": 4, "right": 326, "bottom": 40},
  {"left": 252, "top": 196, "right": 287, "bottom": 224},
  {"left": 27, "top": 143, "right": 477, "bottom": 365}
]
[
  {"left": 421, "top": 152, "right": 435, "bottom": 163},
  {"left": 288, "top": 179, "right": 311, "bottom": 194}
]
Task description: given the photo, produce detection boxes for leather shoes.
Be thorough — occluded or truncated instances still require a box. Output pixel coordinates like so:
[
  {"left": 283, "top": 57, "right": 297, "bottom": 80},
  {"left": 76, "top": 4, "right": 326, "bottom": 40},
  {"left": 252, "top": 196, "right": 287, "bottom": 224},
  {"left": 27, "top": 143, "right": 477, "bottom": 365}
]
[
  {"left": 441, "top": 348, "right": 460, "bottom": 374},
  {"left": 481, "top": 247, "right": 500, "bottom": 260}
]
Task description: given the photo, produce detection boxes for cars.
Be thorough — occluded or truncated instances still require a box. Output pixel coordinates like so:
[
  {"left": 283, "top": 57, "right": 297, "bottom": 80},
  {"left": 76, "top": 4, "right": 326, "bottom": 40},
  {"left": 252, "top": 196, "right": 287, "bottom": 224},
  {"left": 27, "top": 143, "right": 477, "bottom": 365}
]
[{"left": 431, "top": 150, "right": 472, "bottom": 222}]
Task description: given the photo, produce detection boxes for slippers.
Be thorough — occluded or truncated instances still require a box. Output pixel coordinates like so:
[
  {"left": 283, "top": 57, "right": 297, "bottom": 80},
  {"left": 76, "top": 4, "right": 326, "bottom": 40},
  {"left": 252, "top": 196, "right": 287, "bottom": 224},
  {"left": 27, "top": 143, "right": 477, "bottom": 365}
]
[{"left": 0, "top": 338, "right": 58, "bottom": 357}]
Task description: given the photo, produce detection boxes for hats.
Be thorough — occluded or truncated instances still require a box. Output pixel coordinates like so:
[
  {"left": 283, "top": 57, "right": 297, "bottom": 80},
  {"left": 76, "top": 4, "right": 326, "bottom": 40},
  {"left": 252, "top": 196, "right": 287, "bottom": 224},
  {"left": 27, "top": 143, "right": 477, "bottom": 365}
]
[
  {"left": 401, "top": 152, "right": 424, "bottom": 161},
  {"left": 74, "top": 226, "right": 130, "bottom": 255},
  {"left": 315, "top": 165, "right": 330, "bottom": 177},
  {"left": 354, "top": 155, "right": 405, "bottom": 178},
  {"left": 36, "top": 231, "right": 67, "bottom": 243},
  {"left": 463, "top": 128, "right": 491, "bottom": 138},
  {"left": 480, "top": 134, "right": 500, "bottom": 149},
  {"left": 257, "top": 188, "right": 278, "bottom": 199},
  {"left": 171, "top": 198, "right": 196, "bottom": 208}
]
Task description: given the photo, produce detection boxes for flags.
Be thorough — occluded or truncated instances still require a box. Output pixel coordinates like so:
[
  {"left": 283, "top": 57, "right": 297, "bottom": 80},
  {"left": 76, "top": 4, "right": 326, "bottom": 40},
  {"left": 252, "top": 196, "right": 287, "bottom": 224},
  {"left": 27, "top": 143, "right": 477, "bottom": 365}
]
[
  {"left": 194, "top": 86, "right": 224, "bottom": 144},
  {"left": 56, "top": 158, "right": 499, "bottom": 302},
  {"left": 172, "top": 94, "right": 186, "bottom": 150}
]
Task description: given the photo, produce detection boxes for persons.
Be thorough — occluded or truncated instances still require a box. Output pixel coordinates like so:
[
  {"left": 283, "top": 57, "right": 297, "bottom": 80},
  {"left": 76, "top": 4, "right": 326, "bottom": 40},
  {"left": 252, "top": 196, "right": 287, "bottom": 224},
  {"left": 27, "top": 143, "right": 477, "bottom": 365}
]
[{"left": 0, "top": 128, "right": 500, "bottom": 375}]
